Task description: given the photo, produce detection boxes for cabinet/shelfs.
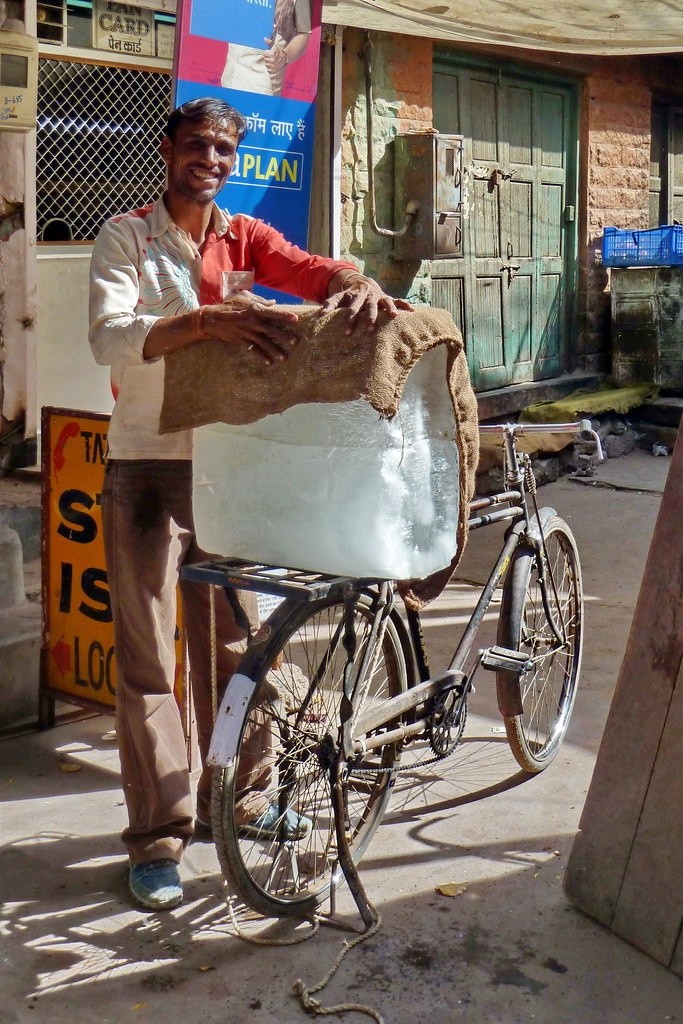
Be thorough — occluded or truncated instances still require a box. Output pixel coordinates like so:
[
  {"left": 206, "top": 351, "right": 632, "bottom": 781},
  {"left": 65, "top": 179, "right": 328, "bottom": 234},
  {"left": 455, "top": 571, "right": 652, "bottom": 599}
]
[
  {"left": 393, "top": 134, "right": 465, "bottom": 260},
  {"left": 609, "top": 267, "right": 683, "bottom": 389}
]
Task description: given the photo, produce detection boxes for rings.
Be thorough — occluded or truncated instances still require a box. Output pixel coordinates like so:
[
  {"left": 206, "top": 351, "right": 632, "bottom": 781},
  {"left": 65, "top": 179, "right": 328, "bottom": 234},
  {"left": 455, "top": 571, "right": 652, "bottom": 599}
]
[{"left": 247, "top": 345, "right": 254, "bottom": 352}]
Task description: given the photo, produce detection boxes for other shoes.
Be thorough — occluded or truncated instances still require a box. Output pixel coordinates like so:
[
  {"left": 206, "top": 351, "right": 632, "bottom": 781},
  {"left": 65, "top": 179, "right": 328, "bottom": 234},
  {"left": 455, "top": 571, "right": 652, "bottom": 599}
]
[
  {"left": 197, "top": 803, "right": 313, "bottom": 839},
  {"left": 130, "top": 857, "right": 184, "bottom": 910}
]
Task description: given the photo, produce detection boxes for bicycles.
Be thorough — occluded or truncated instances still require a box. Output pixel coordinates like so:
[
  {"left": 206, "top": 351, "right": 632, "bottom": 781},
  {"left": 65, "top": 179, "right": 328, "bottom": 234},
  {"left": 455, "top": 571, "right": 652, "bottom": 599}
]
[{"left": 176, "top": 419, "right": 609, "bottom": 935}]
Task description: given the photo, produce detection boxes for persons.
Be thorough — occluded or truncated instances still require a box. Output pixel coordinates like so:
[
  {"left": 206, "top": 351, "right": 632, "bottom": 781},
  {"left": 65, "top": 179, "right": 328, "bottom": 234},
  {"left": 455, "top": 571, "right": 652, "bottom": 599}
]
[
  {"left": 88, "top": 97, "right": 415, "bottom": 911},
  {"left": 221, "top": 0, "right": 312, "bottom": 96}
]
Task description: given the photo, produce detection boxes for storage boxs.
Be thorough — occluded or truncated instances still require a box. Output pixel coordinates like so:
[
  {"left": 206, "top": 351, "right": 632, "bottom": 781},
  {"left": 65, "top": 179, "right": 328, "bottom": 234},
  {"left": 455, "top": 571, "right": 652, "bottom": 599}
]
[{"left": 604, "top": 223, "right": 683, "bottom": 267}]
